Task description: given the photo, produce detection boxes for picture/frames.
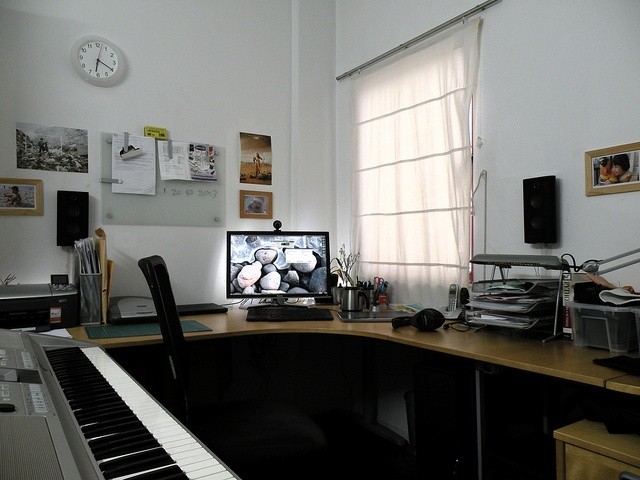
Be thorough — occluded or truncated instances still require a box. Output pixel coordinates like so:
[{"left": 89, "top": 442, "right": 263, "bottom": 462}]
[
  {"left": 585, "top": 142, "right": 640, "bottom": 196},
  {"left": 240, "top": 190, "right": 273, "bottom": 219},
  {"left": 0, "top": 178, "right": 44, "bottom": 216}
]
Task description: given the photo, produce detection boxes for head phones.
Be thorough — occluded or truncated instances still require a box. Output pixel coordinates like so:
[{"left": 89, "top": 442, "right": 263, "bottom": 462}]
[{"left": 392, "top": 308, "right": 445, "bottom": 330}]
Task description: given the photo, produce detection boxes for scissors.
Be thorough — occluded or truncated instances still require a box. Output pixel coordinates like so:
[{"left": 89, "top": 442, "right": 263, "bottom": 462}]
[{"left": 374, "top": 277, "right": 384, "bottom": 289}]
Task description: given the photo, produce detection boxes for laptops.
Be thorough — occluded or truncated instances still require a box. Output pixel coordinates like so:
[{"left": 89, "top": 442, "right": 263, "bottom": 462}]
[{"left": 176, "top": 303, "right": 228, "bottom": 316}]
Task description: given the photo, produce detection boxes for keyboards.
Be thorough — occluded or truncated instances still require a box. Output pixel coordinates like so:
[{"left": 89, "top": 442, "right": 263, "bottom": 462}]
[{"left": 245, "top": 306, "right": 333, "bottom": 322}]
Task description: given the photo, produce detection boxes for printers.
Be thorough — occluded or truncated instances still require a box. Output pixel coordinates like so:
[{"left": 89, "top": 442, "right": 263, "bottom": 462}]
[{"left": 0, "top": 284, "right": 79, "bottom": 332}]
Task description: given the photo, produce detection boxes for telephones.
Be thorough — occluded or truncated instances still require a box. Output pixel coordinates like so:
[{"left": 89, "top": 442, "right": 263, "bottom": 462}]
[{"left": 438, "top": 284, "right": 462, "bottom": 320}]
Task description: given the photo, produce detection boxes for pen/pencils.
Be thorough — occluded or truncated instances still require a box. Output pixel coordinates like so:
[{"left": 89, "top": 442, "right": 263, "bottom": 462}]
[{"left": 355, "top": 274, "right": 373, "bottom": 289}]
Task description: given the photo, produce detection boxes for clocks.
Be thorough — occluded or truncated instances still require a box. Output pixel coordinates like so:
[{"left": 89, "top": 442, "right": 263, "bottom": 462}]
[{"left": 70, "top": 35, "right": 128, "bottom": 87}]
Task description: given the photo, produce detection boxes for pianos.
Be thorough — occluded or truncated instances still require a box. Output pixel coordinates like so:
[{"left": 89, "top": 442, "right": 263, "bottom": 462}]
[{"left": 0, "top": 330, "right": 239, "bottom": 479}]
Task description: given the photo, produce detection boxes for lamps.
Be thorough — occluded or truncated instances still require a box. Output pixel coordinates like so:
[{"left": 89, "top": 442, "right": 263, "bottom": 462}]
[{"left": 471, "top": 248, "right": 640, "bottom": 278}]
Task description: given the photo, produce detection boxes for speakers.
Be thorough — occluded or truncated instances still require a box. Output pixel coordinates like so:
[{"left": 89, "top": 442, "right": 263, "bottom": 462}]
[
  {"left": 521, "top": 175, "right": 558, "bottom": 243},
  {"left": 56, "top": 189, "right": 90, "bottom": 247}
]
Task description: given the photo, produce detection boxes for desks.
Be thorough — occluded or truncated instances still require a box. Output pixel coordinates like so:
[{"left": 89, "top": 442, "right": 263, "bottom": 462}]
[{"left": 68, "top": 310, "right": 639, "bottom": 478}]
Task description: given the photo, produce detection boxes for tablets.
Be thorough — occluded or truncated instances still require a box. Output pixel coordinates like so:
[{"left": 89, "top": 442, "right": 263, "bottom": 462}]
[{"left": 339, "top": 310, "right": 415, "bottom": 322}]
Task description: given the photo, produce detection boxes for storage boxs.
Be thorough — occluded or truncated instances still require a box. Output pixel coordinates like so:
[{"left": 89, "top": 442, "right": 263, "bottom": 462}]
[
  {"left": 565, "top": 301, "right": 631, "bottom": 353},
  {"left": 632, "top": 309, "right": 639, "bottom": 351}
]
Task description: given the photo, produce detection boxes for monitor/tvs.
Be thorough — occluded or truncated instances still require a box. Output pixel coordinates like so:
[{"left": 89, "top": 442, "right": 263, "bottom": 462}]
[{"left": 226, "top": 231, "right": 331, "bottom": 309}]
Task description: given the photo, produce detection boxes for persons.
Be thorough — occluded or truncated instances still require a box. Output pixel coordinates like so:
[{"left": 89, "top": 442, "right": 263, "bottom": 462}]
[
  {"left": 253, "top": 152, "right": 262, "bottom": 176},
  {"left": 9, "top": 186, "right": 22, "bottom": 205},
  {"left": 37, "top": 137, "right": 45, "bottom": 152},
  {"left": 612, "top": 153, "right": 632, "bottom": 182},
  {"left": 245, "top": 197, "right": 268, "bottom": 213}
]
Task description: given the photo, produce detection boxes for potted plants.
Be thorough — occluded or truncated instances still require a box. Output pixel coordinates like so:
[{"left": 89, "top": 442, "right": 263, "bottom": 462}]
[{"left": 330, "top": 243, "right": 360, "bottom": 305}]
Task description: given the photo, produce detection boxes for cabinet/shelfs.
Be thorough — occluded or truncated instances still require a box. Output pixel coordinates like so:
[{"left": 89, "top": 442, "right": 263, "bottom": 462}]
[{"left": 553, "top": 419, "right": 640, "bottom": 479}]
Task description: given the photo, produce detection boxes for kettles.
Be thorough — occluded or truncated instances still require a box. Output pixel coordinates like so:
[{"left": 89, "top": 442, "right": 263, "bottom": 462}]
[{"left": 342, "top": 287, "right": 370, "bottom": 312}]
